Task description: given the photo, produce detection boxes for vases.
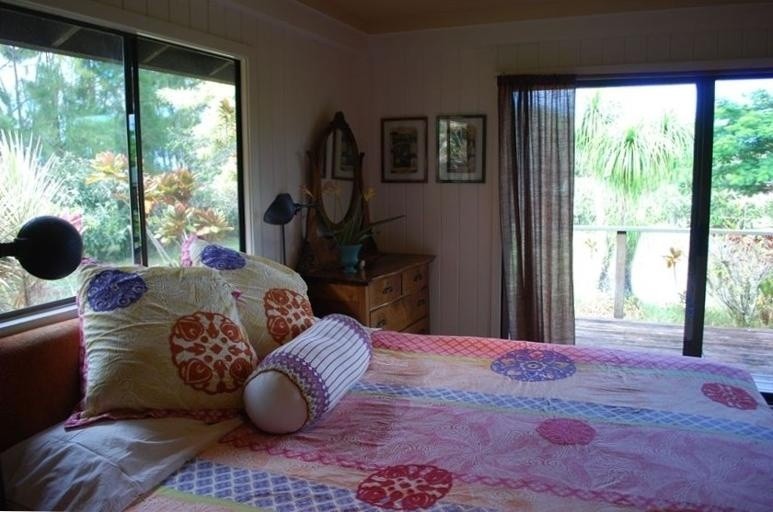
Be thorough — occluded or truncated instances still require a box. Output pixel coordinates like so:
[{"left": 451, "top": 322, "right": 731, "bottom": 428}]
[{"left": 333, "top": 245, "right": 363, "bottom": 273}]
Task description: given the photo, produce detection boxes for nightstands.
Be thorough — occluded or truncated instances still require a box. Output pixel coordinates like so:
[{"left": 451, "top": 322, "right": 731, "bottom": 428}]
[{"left": 302, "top": 251, "right": 434, "bottom": 334}]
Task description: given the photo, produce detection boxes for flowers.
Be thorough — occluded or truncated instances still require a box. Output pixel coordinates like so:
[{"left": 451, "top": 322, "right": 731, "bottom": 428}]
[{"left": 299, "top": 179, "right": 380, "bottom": 245}]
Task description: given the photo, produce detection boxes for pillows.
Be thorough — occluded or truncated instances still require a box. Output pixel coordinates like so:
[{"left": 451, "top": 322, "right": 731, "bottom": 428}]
[
  {"left": 64, "top": 255, "right": 266, "bottom": 433},
  {"left": 244, "top": 314, "right": 372, "bottom": 434},
  {"left": 181, "top": 232, "right": 317, "bottom": 360}
]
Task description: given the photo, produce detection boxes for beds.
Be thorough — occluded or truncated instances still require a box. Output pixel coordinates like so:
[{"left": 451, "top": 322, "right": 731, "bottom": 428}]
[{"left": 0, "top": 314, "right": 772, "bottom": 511}]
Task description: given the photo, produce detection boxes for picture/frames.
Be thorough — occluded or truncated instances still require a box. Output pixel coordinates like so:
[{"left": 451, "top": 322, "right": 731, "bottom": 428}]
[
  {"left": 319, "top": 130, "right": 353, "bottom": 182},
  {"left": 379, "top": 114, "right": 486, "bottom": 184}
]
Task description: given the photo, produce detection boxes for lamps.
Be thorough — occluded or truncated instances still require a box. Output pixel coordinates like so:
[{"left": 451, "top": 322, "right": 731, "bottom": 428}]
[{"left": 263, "top": 193, "right": 319, "bottom": 265}]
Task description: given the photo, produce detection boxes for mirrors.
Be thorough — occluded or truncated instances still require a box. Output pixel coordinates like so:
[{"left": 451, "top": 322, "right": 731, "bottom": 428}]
[{"left": 310, "top": 108, "right": 363, "bottom": 234}]
